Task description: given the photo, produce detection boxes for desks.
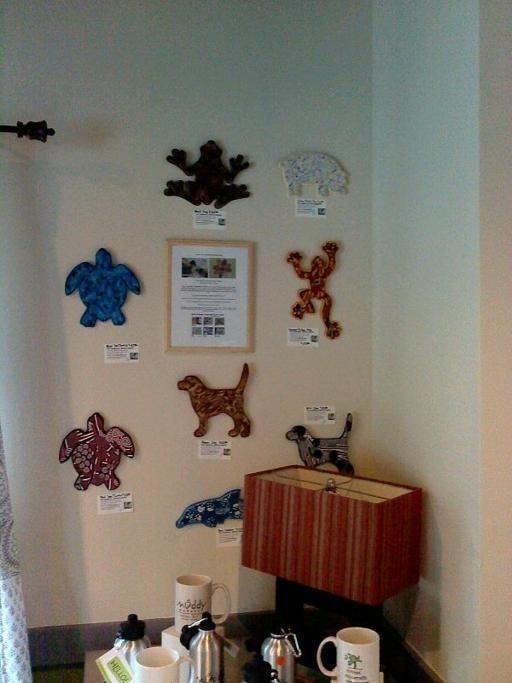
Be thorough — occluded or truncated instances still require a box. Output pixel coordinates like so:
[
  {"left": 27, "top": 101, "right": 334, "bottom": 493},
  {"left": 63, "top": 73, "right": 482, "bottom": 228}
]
[{"left": 82, "top": 604, "right": 432, "bottom": 683}]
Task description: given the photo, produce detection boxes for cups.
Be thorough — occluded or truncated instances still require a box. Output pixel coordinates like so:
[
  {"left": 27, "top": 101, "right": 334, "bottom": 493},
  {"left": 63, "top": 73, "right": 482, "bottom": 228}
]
[
  {"left": 316, "top": 627, "right": 380, "bottom": 682},
  {"left": 174, "top": 574, "right": 233, "bottom": 634},
  {"left": 239, "top": 658, "right": 280, "bottom": 682},
  {"left": 133, "top": 646, "right": 198, "bottom": 682}
]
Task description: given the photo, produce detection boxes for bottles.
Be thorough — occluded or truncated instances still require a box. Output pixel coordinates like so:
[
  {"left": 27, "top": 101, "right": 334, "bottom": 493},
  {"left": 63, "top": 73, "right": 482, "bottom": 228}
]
[
  {"left": 189, "top": 610, "right": 225, "bottom": 682},
  {"left": 113, "top": 611, "right": 152, "bottom": 670},
  {"left": 261, "top": 628, "right": 301, "bottom": 682}
]
